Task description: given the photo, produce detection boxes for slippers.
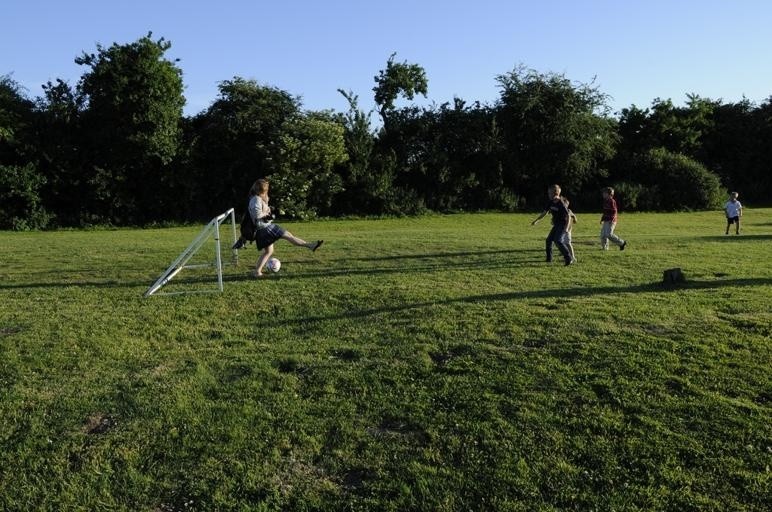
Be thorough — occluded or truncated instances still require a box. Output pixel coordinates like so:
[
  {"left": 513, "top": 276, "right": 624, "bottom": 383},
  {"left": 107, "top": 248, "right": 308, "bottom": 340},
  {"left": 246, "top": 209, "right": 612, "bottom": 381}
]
[{"left": 312, "top": 238, "right": 324, "bottom": 252}]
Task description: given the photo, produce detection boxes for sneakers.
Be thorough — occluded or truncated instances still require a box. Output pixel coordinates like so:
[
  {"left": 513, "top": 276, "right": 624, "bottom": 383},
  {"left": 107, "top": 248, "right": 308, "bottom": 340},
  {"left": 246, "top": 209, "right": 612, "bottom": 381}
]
[{"left": 544, "top": 240, "right": 627, "bottom": 267}]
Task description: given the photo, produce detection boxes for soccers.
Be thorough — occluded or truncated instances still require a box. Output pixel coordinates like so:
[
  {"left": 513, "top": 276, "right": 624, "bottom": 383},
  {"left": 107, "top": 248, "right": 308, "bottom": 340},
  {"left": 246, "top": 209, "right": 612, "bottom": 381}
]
[{"left": 265, "top": 258, "right": 281, "bottom": 274}]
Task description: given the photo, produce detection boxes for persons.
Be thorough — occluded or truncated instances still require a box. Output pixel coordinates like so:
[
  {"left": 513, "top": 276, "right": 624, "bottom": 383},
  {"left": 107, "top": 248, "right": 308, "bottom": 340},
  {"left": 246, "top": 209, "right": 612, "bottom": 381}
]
[
  {"left": 246, "top": 177, "right": 325, "bottom": 279},
  {"left": 723, "top": 191, "right": 743, "bottom": 236},
  {"left": 559, "top": 196, "right": 579, "bottom": 264},
  {"left": 599, "top": 184, "right": 627, "bottom": 251},
  {"left": 531, "top": 183, "right": 573, "bottom": 267}
]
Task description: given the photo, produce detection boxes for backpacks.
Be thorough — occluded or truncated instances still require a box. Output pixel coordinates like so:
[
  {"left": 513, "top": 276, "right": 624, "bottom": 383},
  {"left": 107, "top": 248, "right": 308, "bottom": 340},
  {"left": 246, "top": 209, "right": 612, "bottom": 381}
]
[{"left": 239, "top": 206, "right": 257, "bottom": 243}]
